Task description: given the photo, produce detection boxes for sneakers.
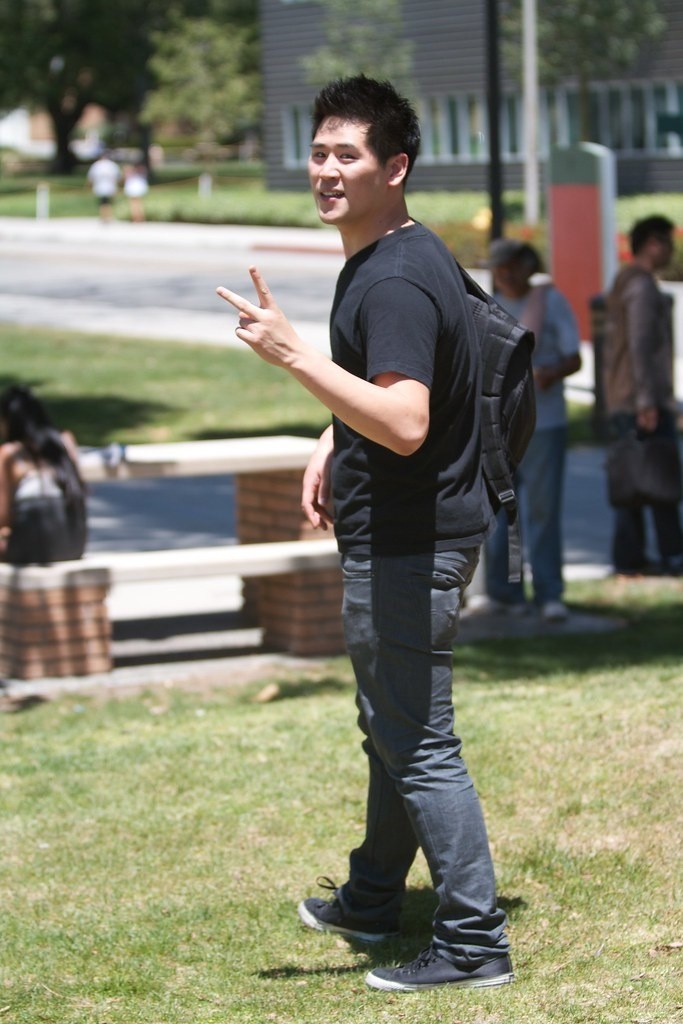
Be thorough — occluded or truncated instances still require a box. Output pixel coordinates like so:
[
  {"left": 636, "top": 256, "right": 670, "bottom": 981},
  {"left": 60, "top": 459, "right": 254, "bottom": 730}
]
[
  {"left": 298, "top": 876, "right": 400, "bottom": 941},
  {"left": 365, "top": 940, "right": 515, "bottom": 990}
]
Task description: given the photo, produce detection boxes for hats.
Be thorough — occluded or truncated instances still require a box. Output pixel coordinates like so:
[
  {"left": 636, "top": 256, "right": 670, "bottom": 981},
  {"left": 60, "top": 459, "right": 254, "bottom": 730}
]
[{"left": 478, "top": 241, "right": 528, "bottom": 269}]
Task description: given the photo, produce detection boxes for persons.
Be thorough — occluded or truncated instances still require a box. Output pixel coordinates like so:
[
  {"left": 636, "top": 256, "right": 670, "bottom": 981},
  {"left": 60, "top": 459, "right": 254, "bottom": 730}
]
[
  {"left": 466, "top": 215, "right": 683, "bottom": 619},
  {"left": 88, "top": 154, "right": 149, "bottom": 219},
  {"left": 0, "top": 372, "right": 87, "bottom": 566},
  {"left": 216, "top": 72, "right": 517, "bottom": 991}
]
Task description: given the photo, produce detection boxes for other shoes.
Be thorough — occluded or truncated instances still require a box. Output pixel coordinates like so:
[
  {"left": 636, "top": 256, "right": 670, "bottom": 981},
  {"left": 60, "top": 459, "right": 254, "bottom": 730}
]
[
  {"left": 542, "top": 599, "right": 566, "bottom": 622},
  {"left": 613, "top": 557, "right": 662, "bottom": 576},
  {"left": 467, "top": 596, "right": 526, "bottom": 614}
]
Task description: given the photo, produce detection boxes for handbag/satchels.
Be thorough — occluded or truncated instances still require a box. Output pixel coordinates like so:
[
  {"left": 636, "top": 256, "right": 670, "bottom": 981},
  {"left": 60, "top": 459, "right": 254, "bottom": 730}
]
[{"left": 605, "top": 427, "right": 682, "bottom": 507}]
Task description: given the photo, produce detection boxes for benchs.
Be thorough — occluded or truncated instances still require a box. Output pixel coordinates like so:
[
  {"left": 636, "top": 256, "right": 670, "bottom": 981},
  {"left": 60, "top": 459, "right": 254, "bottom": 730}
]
[
  {"left": 0, "top": 435, "right": 335, "bottom": 619},
  {"left": 0, "top": 538, "right": 346, "bottom": 682}
]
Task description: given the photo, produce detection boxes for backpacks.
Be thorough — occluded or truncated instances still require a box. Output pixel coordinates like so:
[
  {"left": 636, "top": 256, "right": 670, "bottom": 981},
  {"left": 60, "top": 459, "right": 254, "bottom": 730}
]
[{"left": 453, "top": 253, "right": 536, "bottom": 527}]
